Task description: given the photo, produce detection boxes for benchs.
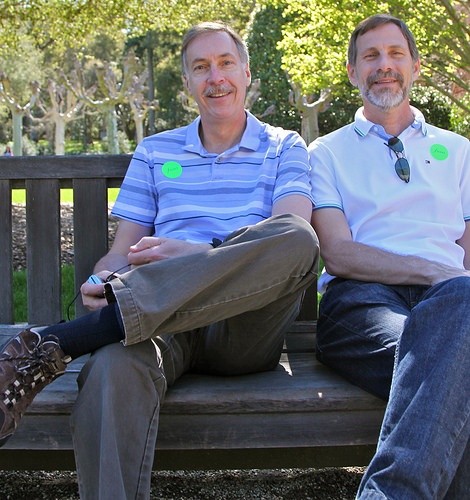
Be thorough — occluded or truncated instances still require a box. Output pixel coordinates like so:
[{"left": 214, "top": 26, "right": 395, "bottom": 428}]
[{"left": 0, "top": 155, "right": 387, "bottom": 449}]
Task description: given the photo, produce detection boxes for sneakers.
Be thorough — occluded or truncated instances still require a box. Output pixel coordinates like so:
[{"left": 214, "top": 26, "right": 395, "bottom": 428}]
[{"left": 1, "top": 324, "right": 73, "bottom": 448}]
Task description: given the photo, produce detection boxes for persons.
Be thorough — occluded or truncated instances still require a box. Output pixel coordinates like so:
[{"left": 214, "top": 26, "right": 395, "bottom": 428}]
[
  {"left": 307, "top": 13, "right": 470, "bottom": 499},
  {"left": 0, "top": 21, "right": 320, "bottom": 500}
]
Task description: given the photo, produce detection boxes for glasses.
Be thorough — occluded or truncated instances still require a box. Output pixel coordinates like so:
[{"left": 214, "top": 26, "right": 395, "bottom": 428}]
[{"left": 384, "top": 136, "right": 411, "bottom": 183}]
